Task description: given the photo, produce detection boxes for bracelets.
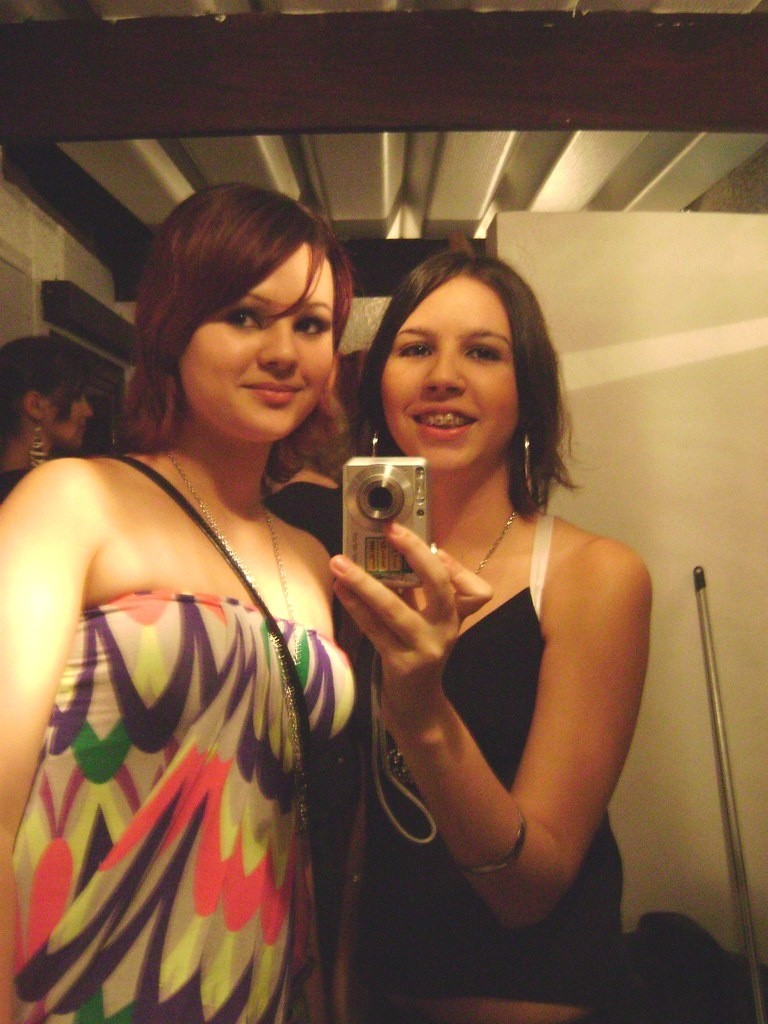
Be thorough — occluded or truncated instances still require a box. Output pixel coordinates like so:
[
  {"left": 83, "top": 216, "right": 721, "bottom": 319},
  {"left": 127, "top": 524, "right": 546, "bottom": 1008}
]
[{"left": 450, "top": 806, "right": 527, "bottom": 875}]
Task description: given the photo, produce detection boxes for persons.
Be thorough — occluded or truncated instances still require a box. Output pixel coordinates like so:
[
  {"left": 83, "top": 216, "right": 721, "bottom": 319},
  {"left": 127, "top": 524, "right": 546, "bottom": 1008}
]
[
  {"left": 0, "top": 183, "right": 357, "bottom": 1022},
  {"left": 261, "top": 472, "right": 342, "bottom": 558},
  {"left": 0, "top": 337, "right": 94, "bottom": 504},
  {"left": 332, "top": 250, "right": 653, "bottom": 1024}
]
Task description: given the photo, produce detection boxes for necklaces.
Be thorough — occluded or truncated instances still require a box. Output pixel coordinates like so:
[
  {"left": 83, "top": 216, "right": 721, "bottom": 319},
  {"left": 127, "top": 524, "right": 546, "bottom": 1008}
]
[
  {"left": 386, "top": 511, "right": 517, "bottom": 785},
  {"left": 164, "top": 452, "right": 310, "bottom": 833}
]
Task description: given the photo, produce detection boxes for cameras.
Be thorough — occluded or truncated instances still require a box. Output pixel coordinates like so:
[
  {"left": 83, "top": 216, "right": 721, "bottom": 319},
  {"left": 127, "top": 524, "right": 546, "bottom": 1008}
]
[{"left": 341, "top": 456, "right": 430, "bottom": 588}]
